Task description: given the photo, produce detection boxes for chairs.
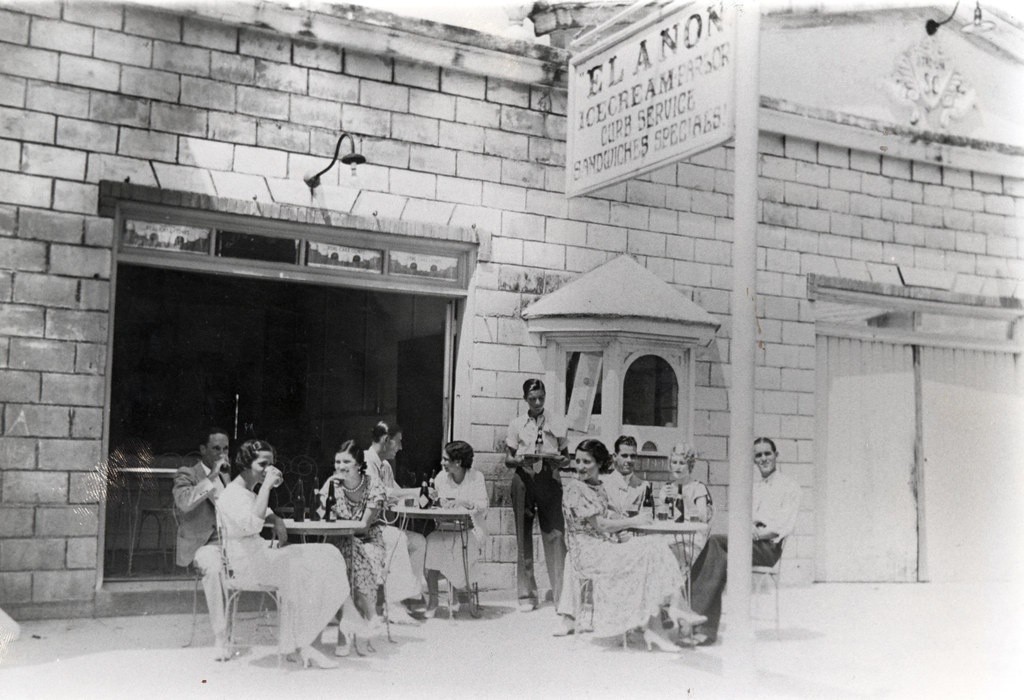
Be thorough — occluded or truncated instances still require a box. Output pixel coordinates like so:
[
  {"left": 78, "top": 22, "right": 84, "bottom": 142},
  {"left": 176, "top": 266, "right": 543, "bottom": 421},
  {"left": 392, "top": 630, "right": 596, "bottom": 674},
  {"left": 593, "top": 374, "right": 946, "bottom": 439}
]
[
  {"left": 568, "top": 541, "right": 634, "bottom": 652},
  {"left": 752, "top": 526, "right": 803, "bottom": 640},
  {"left": 216, "top": 510, "right": 284, "bottom": 664},
  {"left": 171, "top": 504, "right": 233, "bottom": 650}
]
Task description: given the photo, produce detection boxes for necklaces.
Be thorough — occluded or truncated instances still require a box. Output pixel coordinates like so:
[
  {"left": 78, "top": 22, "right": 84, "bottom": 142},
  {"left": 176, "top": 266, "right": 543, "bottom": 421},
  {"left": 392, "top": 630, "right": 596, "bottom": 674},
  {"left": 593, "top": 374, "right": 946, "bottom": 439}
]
[{"left": 342, "top": 475, "right": 364, "bottom": 492}]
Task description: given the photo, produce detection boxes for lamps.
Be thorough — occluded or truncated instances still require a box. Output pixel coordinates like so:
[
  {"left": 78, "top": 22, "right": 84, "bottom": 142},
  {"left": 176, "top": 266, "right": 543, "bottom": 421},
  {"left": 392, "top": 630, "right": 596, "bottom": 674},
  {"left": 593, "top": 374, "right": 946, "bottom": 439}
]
[
  {"left": 927, "top": 0, "right": 996, "bottom": 37},
  {"left": 304, "top": 131, "right": 365, "bottom": 189}
]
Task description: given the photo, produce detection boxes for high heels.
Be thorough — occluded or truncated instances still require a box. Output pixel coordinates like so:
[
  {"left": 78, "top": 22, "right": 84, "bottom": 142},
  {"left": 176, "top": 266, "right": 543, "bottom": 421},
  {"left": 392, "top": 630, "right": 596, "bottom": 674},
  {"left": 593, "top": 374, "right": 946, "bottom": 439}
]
[
  {"left": 669, "top": 607, "right": 707, "bottom": 628},
  {"left": 644, "top": 628, "right": 683, "bottom": 653},
  {"left": 301, "top": 648, "right": 338, "bottom": 668},
  {"left": 339, "top": 619, "right": 377, "bottom": 643}
]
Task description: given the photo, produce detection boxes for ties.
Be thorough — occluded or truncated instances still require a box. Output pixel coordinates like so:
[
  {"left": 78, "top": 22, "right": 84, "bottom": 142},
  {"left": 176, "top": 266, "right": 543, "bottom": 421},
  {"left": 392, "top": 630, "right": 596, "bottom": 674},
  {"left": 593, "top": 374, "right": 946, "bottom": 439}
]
[{"left": 531, "top": 419, "right": 545, "bottom": 474}]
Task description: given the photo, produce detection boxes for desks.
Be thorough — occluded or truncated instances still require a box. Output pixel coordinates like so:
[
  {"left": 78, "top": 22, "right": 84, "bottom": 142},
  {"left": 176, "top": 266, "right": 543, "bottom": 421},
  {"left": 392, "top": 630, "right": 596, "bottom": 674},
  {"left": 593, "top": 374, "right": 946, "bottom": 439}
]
[
  {"left": 270, "top": 520, "right": 365, "bottom": 647},
  {"left": 628, "top": 521, "right": 710, "bottom": 652},
  {"left": 386, "top": 505, "right": 478, "bottom": 615},
  {"left": 114, "top": 466, "right": 178, "bottom": 575}
]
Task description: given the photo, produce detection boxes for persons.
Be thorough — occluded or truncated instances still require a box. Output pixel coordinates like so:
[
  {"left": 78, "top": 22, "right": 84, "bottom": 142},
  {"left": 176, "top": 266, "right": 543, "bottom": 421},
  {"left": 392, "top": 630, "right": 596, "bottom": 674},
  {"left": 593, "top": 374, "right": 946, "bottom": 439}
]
[
  {"left": 171, "top": 429, "right": 289, "bottom": 661},
  {"left": 677, "top": 435, "right": 800, "bottom": 646},
  {"left": 423, "top": 441, "right": 489, "bottom": 619},
  {"left": 554, "top": 436, "right": 656, "bottom": 636},
  {"left": 658, "top": 443, "right": 711, "bottom": 525},
  {"left": 560, "top": 439, "right": 707, "bottom": 654},
  {"left": 362, "top": 417, "right": 428, "bottom": 625},
  {"left": 317, "top": 438, "right": 387, "bottom": 658},
  {"left": 503, "top": 379, "right": 568, "bottom": 611},
  {"left": 216, "top": 435, "right": 376, "bottom": 667}
]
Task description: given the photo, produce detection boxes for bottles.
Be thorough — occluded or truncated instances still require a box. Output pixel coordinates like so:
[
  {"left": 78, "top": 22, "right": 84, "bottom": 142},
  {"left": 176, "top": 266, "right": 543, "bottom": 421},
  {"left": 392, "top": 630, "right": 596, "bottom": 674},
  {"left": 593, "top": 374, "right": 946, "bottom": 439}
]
[
  {"left": 308, "top": 477, "right": 320, "bottom": 520},
  {"left": 642, "top": 482, "right": 655, "bottom": 520},
  {"left": 428, "top": 469, "right": 436, "bottom": 491},
  {"left": 418, "top": 473, "right": 429, "bottom": 510},
  {"left": 664, "top": 481, "right": 674, "bottom": 516},
  {"left": 534, "top": 426, "right": 544, "bottom": 454},
  {"left": 674, "top": 484, "right": 684, "bottom": 523},
  {"left": 325, "top": 480, "right": 337, "bottom": 521},
  {"left": 292, "top": 481, "right": 306, "bottom": 522}
]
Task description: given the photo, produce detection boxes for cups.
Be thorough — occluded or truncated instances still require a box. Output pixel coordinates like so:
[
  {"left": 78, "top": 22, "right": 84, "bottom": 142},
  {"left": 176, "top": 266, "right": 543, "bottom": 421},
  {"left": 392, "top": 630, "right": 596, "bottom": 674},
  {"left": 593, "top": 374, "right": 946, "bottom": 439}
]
[
  {"left": 688, "top": 508, "right": 701, "bottom": 522},
  {"left": 264, "top": 473, "right": 281, "bottom": 487},
  {"left": 657, "top": 506, "right": 669, "bottom": 521},
  {"left": 218, "top": 454, "right": 229, "bottom": 472}
]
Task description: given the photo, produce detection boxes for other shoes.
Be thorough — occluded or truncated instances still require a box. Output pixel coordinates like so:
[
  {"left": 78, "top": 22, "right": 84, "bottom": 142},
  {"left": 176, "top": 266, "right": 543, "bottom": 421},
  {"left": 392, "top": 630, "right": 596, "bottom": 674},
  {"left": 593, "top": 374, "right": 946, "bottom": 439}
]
[
  {"left": 424, "top": 603, "right": 438, "bottom": 618},
  {"left": 520, "top": 604, "right": 534, "bottom": 612},
  {"left": 381, "top": 601, "right": 421, "bottom": 626},
  {"left": 448, "top": 599, "right": 462, "bottom": 612},
  {"left": 215, "top": 643, "right": 232, "bottom": 660},
  {"left": 553, "top": 620, "right": 574, "bottom": 636}
]
